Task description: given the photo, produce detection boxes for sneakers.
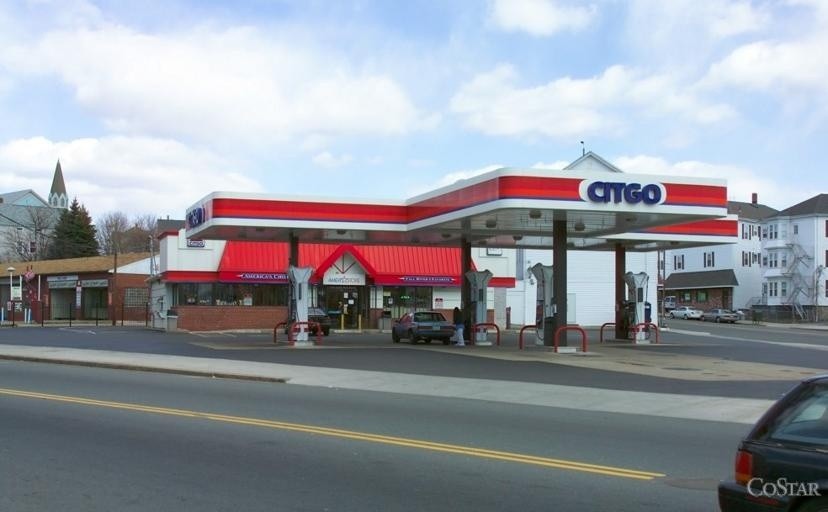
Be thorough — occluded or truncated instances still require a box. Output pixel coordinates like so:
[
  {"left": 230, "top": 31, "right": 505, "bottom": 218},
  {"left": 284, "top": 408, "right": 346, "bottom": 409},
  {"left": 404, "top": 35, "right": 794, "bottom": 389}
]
[{"left": 455, "top": 343, "right": 464, "bottom": 346}]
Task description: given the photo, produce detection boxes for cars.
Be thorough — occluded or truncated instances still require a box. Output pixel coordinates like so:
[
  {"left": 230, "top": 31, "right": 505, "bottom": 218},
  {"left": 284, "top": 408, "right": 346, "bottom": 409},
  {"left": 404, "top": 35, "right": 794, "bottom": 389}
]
[
  {"left": 669, "top": 305, "right": 705, "bottom": 320},
  {"left": 322, "top": 306, "right": 348, "bottom": 328},
  {"left": 700, "top": 307, "right": 739, "bottom": 324},
  {"left": 393, "top": 310, "right": 456, "bottom": 345},
  {"left": 717, "top": 374, "right": 828, "bottom": 511},
  {"left": 286, "top": 307, "right": 334, "bottom": 336}
]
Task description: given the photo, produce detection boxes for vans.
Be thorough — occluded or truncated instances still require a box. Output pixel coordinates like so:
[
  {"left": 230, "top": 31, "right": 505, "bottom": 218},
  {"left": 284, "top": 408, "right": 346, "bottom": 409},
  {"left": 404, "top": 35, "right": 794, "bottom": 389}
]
[{"left": 664, "top": 295, "right": 676, "bottom": 312}]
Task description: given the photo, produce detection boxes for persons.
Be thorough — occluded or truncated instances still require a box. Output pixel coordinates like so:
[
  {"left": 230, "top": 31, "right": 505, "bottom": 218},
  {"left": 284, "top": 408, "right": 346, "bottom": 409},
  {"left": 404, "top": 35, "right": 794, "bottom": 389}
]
[{"left": 452, "top": 305, "right": 467, "bottom": 347}]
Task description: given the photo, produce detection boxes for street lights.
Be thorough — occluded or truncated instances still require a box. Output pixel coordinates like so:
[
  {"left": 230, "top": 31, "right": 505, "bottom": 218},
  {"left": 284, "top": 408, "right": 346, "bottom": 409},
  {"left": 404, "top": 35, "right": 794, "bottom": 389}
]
[{"left": 5, "top": 266, "right": 15, "bottom": 302}]
